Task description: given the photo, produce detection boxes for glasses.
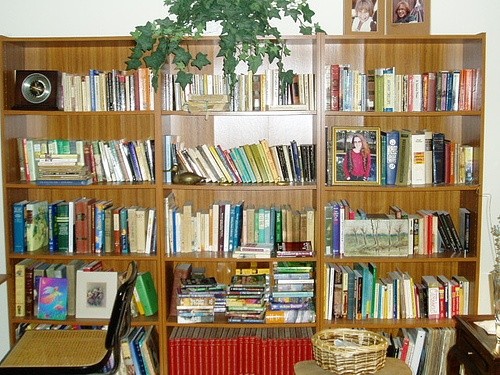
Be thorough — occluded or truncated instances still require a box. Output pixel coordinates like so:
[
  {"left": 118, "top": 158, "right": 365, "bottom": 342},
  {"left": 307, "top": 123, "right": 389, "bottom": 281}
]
[{"left": 353, "top": 141, "right": 362, "bottom": 143}]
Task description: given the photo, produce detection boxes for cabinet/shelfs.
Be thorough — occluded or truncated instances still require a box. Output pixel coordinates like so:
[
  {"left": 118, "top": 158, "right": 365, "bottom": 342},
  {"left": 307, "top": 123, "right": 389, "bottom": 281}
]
[{"left": 0, "top": 33, "right": 488, "bottom": 375}]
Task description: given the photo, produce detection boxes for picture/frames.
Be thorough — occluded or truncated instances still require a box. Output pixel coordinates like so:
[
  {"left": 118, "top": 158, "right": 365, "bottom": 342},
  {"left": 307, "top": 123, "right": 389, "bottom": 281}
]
[
  {"left": 332, "top": 127, "right": 380, "bottom": 186},
  {"left": 76, "top": 270, "right": 117, "bottom": 320},
  {"left": 343, "top": 0, "right": 384, "bottom": 35},
  {"left": 386, "top": 0, "right": 431, "bottom": 36}
]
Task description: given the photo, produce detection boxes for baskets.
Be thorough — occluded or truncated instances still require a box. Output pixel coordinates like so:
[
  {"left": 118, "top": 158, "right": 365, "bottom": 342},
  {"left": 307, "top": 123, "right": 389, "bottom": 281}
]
[{"left": 311, "top": 328, "right": 389, "bottom": 375}]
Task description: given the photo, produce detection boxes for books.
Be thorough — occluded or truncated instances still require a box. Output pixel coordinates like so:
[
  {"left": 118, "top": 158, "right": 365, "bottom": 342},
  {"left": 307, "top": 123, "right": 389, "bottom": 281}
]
[{"left": 9, "top": 65, "right": 484, "bottom": 375}]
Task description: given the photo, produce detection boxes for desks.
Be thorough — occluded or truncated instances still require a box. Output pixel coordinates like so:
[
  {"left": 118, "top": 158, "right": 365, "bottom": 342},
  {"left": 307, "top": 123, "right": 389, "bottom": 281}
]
[
  {"left": 293, "top": 358, "right": 412, "bottom": 375},
  {"left": 446, "top": 315, "right": 500, "bottom": 375}
]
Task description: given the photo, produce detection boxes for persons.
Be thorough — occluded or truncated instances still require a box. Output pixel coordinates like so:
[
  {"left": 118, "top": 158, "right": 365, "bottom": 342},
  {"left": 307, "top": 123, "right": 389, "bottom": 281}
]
[
  {"left": 394, "top": 1, "right": 417, "bottom": 24},
  {"left": 352, "top": 0, "right": 377, "bottom": 31}
]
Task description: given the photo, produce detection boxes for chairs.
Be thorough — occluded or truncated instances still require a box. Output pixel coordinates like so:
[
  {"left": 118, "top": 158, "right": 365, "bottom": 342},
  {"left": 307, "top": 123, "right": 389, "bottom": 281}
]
[{"left": 0, "top": 260, "right": 138, "bottom": 375}]
